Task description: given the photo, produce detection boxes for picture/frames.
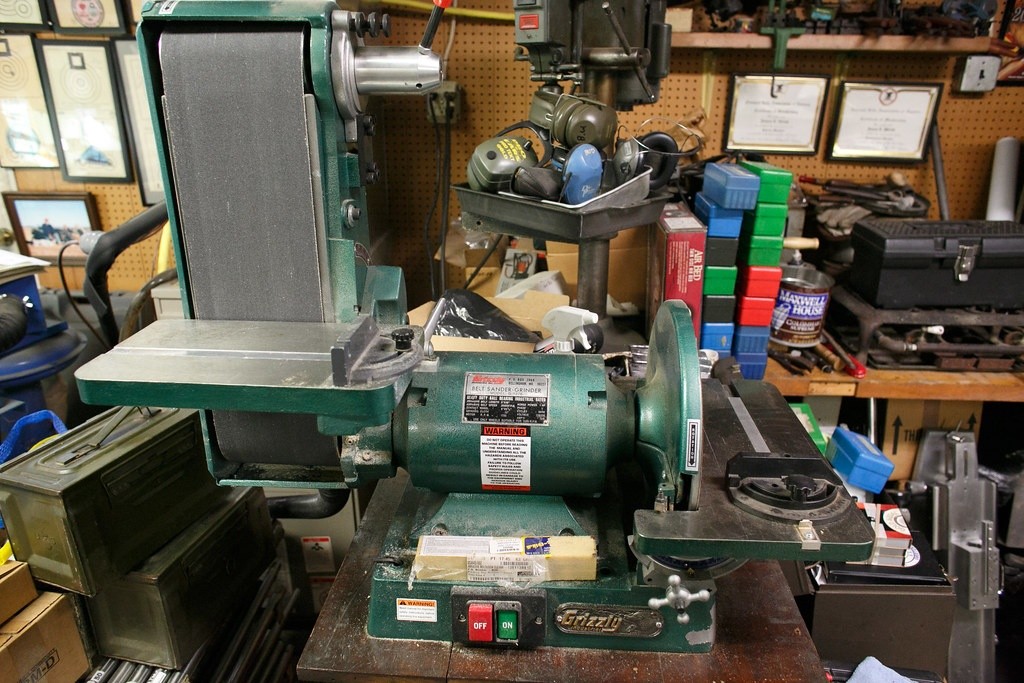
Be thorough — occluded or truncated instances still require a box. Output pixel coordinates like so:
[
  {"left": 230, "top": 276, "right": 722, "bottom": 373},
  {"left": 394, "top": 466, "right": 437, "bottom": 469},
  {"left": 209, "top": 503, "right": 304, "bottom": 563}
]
[
  {"left": 0, "top": 32, "right": 60, "bottom": 169},
  {"left": 109, "top": 37, "right": 167, "bottom": 207},
  {"left": 33, "top": 38, "right": 136, "bottom": 186},
  {"left": 1, "top": 191, "right": 102, "bottom": 269},
  {"left": 45, "top": 0, "right": 131, "bottom": 38},
  {"left": 0, "top": 0, "right": 53, "bottom": 33}
]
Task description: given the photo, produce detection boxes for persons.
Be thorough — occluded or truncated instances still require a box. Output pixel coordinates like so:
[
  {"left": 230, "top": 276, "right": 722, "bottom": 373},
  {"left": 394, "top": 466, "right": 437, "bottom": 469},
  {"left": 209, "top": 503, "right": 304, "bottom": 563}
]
[{"left": 31, "top": 218, "right": 85, "bottom": 245}]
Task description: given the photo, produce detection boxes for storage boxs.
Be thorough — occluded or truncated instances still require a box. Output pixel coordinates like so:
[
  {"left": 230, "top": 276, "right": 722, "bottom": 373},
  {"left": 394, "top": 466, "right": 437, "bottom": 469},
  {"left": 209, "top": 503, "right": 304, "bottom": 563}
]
[
  {"left": 706, "top": 237, "right": 740, "bottom": 266},
  {"left": 847, "top": 218, "right": 1024, "bottom": 313},
  {"left": 0, "top": 559, "right": 39, "bottom": 626},
  {"left": 701, "top": 296, "right": 778, "bottom": 380},
  {"left": 405, "top": 290, "right": 570, "bottom": 355},
  {"left": 883, "top": 400, "right": 985, "bottom": 483},
  {"left": 739, "top": 267, "right": 783, "bottom": 299},
  {"left": 0, "top": 591, "right": 89, "bottom": 683},
  {"left": 738, "top": 160, "right": 793, "bottom": 205},
  {"left": 0, "top": 406, "right": 227, "bottom": 598},
  {"left": 694, "top": 192, "right": 744, "bottom": 238},
  {"left": 702, "top": 162, "right": 761, "bottom": 211},
  {"left": 742, "top": 202, "right": 789, "bottom": 236},
  {"left": 740, "top": 235, "right": 786, "bottom": 267},
  {"left": 83, "top": 484, "right": 278, "bottom": 671},
  {"left": 703, "top": 265, "right": 739, "bottom": 296},
  {"left": 435, "top": 226, "right": 503, "bottom": 300},
  {"left": 642, "top": 202, "right": 707, "bottom": 350},
  {"left": 825, "top": 427, "right": 895, "bottom": 494}
]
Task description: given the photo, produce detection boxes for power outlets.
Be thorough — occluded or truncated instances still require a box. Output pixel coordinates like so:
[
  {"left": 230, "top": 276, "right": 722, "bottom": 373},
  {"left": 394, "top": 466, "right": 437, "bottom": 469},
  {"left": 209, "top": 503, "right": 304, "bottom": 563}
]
[
  {"left": 951, "top": 53, "right": 1003, "bottom": 95},
  {"left": 425, "top": 81, "right": 461, "bottom": 125}
]
